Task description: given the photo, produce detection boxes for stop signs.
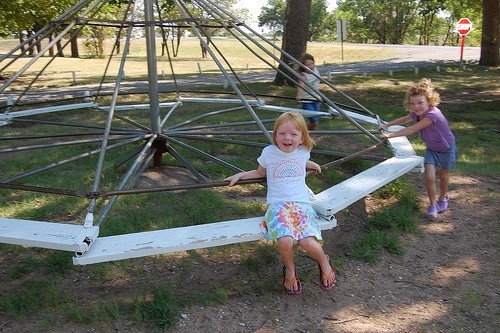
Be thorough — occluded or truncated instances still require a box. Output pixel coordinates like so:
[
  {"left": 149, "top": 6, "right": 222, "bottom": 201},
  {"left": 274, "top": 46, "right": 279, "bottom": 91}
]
[{"left": 456, "top": 18, "right": 472, "bottom": 36}]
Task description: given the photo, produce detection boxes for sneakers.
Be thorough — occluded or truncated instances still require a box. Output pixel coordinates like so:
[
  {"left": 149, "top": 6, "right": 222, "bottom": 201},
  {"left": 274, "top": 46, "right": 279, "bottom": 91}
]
[
  {"left": 425, "top": 205, "right": 438, "bottom": 218},
  {"left": 437, "top": 195, "right": 448, "bottom": 213}
]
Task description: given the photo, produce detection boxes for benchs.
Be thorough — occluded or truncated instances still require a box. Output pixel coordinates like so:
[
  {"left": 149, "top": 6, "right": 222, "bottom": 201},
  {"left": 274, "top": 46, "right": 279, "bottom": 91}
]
[{"left": 0, "top": 97, "right": 424, "bottom": 266}]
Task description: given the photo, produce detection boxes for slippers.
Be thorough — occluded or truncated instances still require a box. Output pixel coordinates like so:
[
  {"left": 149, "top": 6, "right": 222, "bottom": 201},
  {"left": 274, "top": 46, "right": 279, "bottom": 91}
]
[
  {"left": 318, "top": 258, "right": 337, "bottom": 290},
  {"left": 282, "top": 264, "right": 301, "bottom": 295}
]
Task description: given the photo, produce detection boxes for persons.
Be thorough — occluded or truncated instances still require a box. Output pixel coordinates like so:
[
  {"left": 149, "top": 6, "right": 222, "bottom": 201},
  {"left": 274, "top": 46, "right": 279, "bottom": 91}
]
[
  {"left": 378, "top": 77, "right": 457, "bottom": 219},
  {"left": 296, "top": 54, "right": 322, "bottom": 131},
  {"left": 224, "top": 111, "right": 337, "bottom": 295}
]
